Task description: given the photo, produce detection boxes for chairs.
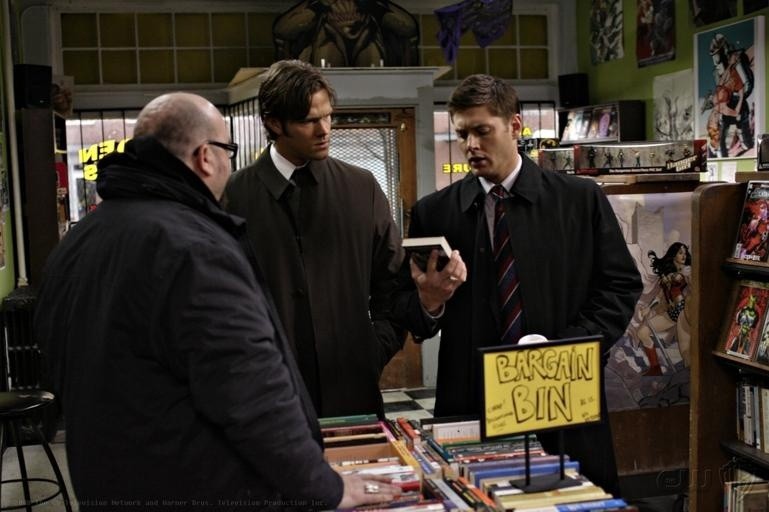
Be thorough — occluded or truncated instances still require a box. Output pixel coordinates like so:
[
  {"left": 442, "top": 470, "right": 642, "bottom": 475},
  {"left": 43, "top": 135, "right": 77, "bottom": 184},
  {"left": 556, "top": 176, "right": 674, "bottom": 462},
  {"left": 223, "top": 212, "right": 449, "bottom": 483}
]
[{"left": 0, "top": 321, "right": 72, "bottom": 512}]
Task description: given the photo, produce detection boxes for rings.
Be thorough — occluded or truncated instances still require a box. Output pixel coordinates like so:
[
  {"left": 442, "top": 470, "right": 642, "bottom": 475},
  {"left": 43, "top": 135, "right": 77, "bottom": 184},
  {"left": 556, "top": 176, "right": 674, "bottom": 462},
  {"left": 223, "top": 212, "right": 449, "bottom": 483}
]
[{"left": 447, "top": 274, "right": 459, "bottom": 282}]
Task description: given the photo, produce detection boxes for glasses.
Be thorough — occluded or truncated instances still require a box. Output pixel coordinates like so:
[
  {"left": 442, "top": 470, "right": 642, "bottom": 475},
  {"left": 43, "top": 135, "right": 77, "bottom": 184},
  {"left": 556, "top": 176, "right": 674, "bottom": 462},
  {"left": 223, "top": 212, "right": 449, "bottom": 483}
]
[{"left": 192, "top": 140, "right": 237, "bottom": 159}]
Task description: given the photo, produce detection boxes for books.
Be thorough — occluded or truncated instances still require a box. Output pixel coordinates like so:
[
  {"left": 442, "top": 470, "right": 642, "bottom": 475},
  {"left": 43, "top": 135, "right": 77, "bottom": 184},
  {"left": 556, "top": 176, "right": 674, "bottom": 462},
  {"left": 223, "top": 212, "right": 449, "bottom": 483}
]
[
  {"left": 721, "top": 380, "right": 769, "bottom": 512},
  {"left": 400, "top": 234, "right": 454, "bottom": 273}
]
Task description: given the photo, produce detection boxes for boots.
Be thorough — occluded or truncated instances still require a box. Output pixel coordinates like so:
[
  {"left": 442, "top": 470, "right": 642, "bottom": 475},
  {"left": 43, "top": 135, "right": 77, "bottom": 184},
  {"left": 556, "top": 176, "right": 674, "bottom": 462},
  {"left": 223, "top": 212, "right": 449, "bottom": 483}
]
[{"left": 641, "top": 342, "right": 662, "bottom": 377}]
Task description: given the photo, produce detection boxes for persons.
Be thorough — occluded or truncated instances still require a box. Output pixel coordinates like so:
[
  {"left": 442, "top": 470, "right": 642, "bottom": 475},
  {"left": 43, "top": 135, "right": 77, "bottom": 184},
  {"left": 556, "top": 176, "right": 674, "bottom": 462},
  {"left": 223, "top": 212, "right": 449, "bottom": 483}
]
[
  {"left": 616, "top": 148, "right": 626, "bottom": 167},
  {"left": 634, "top": 240, "right": 693, "bottom": 377},
  {"left": 602, "top": 150, "right": 614, "bottom": 167},
  {"left": 31, "top": 86, "right": 404, "bottom": 512},
  {"left": 403, "top": 70, "right": 646, "bottom": 500},
  {"left": 648, "top": 149, "right": 656, "bottom": 166},
  {"left": 548, "top": 152, "right": 558, "bottom": 173},
  {"left": 562, "top": 155, "right": 573, "bottom": 171},
  {"left": 683, "top": 145, "right": 691, "bottom": 159},
  {"left": 663, "top": 146, "right": 675, "bottom": 161},
  {"left": 218, "top": 54, "right": 410, "bottom": 419},
  {"left": 586, "top": 144, "right": 596, "bottom": 169},
  {"left": 700, "top": 43, "right": 756, "bottom": 153},
  {"left": 633, "top": 149, "right": 642, "bottom": 168}
]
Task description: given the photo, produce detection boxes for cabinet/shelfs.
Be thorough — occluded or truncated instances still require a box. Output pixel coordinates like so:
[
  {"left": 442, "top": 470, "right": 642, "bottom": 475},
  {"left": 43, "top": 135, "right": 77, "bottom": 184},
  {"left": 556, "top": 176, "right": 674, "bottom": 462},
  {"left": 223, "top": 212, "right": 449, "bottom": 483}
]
[
  {"left": 589, "top": 175, "right": 694, "bottom": 480},
  {"left": 689, "top": 170, "right": 769, "bottom": 512},
  {"left": 22, "top": 110, "right": 72, "bottom": 286}
]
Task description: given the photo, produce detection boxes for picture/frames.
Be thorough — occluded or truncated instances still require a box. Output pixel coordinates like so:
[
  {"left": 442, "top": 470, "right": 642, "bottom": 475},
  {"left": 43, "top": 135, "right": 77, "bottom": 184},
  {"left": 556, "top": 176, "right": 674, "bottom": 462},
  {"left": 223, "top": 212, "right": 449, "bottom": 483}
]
[{"left": 693, "top": 15, "right": 765, "bottom": 161}]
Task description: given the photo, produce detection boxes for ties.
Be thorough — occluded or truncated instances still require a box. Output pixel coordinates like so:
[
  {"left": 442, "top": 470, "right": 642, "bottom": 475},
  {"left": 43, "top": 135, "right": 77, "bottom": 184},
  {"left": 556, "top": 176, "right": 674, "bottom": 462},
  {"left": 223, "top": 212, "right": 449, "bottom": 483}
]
[{"left": 489, "top": 186, "right": 524, "bottom": 344}]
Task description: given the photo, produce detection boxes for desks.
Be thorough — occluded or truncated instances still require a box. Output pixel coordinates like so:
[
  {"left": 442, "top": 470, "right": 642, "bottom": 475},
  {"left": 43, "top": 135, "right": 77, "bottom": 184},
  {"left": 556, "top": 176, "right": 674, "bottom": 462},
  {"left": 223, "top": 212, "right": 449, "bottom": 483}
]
[{"left": 310, "top": 386, "right": 639, "bottom": 512}]
